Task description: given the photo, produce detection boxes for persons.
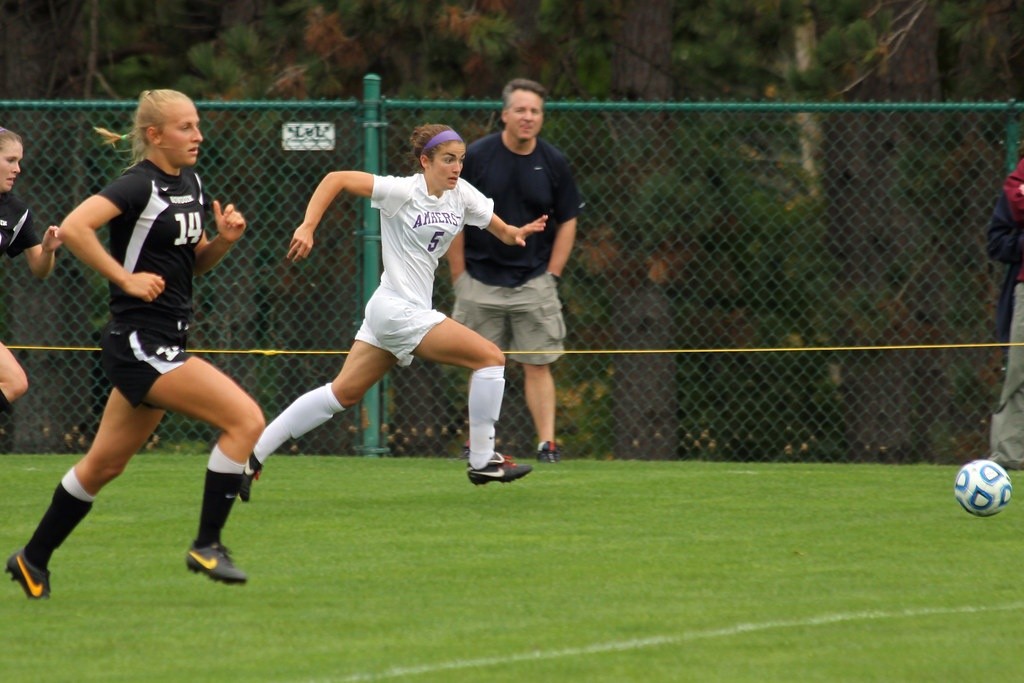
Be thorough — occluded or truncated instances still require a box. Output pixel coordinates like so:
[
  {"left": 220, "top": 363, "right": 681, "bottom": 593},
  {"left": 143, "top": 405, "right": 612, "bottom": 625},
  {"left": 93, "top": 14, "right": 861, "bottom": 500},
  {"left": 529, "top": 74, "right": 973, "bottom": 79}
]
[
  {"left": 240, "top": 123, "right": 548, "bottom": 503},
  {"left": 5, "top": 89, "right": 265, "bottom": 599},
  {"left": 448, "top": 79, "right": 582, "bottom": 464},
  {"left": 986, "top": 157, "right": 1024, "bottom": 471},
  {"left": 0, "top": 126, "right": 63, "bottom": 410}
]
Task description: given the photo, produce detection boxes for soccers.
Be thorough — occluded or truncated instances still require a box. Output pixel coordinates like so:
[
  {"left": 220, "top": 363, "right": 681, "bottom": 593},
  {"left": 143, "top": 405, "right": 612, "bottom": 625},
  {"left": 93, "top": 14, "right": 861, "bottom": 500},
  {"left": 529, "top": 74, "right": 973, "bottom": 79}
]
[{"left": 952, "top": 458, "right": 1014, "bottom": 518}]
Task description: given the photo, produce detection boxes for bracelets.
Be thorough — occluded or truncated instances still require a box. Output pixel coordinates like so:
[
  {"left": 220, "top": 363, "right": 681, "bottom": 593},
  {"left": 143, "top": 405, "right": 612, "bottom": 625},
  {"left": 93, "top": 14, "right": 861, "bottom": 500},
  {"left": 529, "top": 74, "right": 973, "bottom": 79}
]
[{"left": 545, "top": 271, "right": 561, "bottom": 282}]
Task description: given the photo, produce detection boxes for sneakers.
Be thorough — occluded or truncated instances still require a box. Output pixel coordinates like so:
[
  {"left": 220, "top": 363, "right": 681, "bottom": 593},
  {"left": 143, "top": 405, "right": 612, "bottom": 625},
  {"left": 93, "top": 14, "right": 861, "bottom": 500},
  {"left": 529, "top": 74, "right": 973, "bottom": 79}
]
[
  {"left": 5, "top": 550, "right": 51, "bottom": 601},
  {"left": 537, "top": 439, "right": 557, "bottom": 466},
  {"left": 466, "top": 453, "right": 532, "bottom": 483},
  {"left": 240, "top": 452, "right": 263, "bottom": 501},
  {"left": 185, "top": 541, "right": 247, "bottom": 586}
]
